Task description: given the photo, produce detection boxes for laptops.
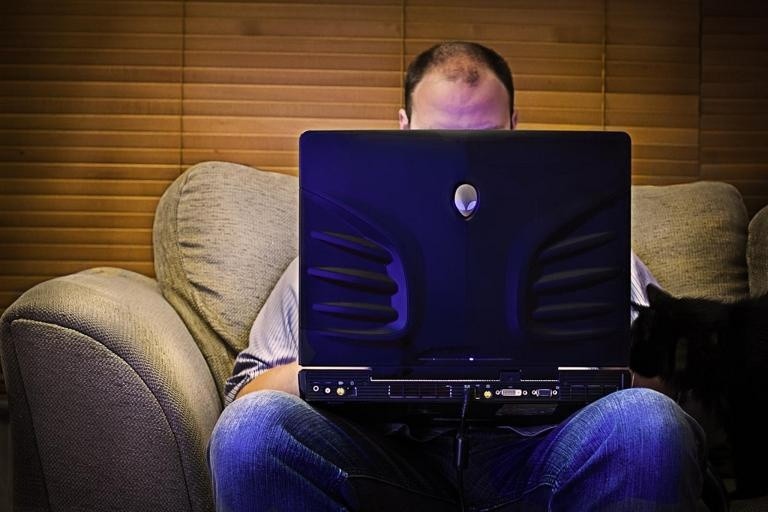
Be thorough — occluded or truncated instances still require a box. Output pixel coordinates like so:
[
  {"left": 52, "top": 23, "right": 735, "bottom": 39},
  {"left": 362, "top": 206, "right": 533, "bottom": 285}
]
[{"left": 299, "top": 131, "right": 631, "bottom": 407}]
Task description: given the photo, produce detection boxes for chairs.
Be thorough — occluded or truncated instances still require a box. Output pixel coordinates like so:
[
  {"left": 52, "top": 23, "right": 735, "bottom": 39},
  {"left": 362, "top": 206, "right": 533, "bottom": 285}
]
[{"left": 0, "top": 158, "right": 751, "bottom": 511}]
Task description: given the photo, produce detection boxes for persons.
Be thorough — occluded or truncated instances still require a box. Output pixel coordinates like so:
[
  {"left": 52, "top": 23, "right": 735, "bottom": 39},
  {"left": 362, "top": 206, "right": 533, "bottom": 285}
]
[{"left": 207, "top": 41, "right": 716, "bottom": 512}]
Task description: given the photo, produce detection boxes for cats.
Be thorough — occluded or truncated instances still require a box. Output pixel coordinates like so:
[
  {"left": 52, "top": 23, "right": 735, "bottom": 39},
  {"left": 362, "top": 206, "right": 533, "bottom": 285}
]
[{"left": 624, "top": 282, "right": 768, "bottom": 499}]
